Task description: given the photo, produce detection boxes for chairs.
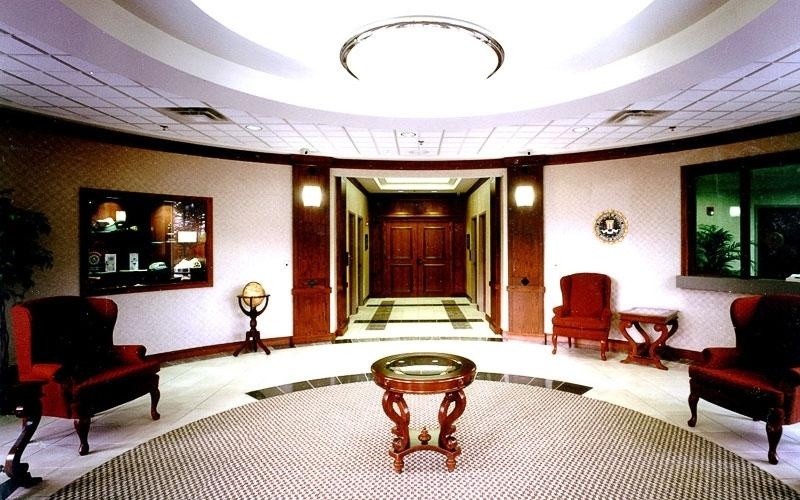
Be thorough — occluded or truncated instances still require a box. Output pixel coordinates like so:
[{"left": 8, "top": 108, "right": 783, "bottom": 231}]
[
  {"left": 552, "top": 273, "right": 613, "bottom": 361},
  {"left": 12, "top": 295, "right": 161, "bottom": 456},
  {"left": 688, "top": 294, "right": 800, "bottom": 465}
]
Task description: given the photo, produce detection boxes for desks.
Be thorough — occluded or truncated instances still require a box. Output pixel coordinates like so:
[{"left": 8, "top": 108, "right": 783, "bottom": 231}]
[{"left": 370, "top": 351, "right": 477, "bottom": 474}]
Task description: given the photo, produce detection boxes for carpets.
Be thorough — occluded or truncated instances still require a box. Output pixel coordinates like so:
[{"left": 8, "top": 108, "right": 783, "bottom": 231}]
[{"left": 45, "top": 378, "right": 800, "bottom": 500}]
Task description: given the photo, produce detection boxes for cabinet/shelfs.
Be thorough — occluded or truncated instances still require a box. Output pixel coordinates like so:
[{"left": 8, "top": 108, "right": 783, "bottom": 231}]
[{"left": 233, "top": 294, "right": 272, "bottom": 356}]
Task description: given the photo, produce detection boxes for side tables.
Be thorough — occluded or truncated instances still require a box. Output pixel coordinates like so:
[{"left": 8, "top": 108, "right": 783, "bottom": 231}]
[
  {"left": 1, "top": 378, "right": 50, "bottom": 500},
  {"left": 618, "top": 307, "right": 680, "bottom": 370}
]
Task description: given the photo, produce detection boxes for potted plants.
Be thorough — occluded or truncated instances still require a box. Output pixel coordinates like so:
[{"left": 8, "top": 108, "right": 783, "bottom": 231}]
[{"left": 0, "top": 188, "right": 54, "bottom": 416}]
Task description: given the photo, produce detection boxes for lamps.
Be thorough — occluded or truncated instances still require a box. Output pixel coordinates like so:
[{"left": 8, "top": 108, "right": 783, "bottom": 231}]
[
  {"left": 302, "top": 186, "right": 321, "bottom": 207},
  {"left": 515, "top": 184, "right": 534, "bottom": 207}
]
